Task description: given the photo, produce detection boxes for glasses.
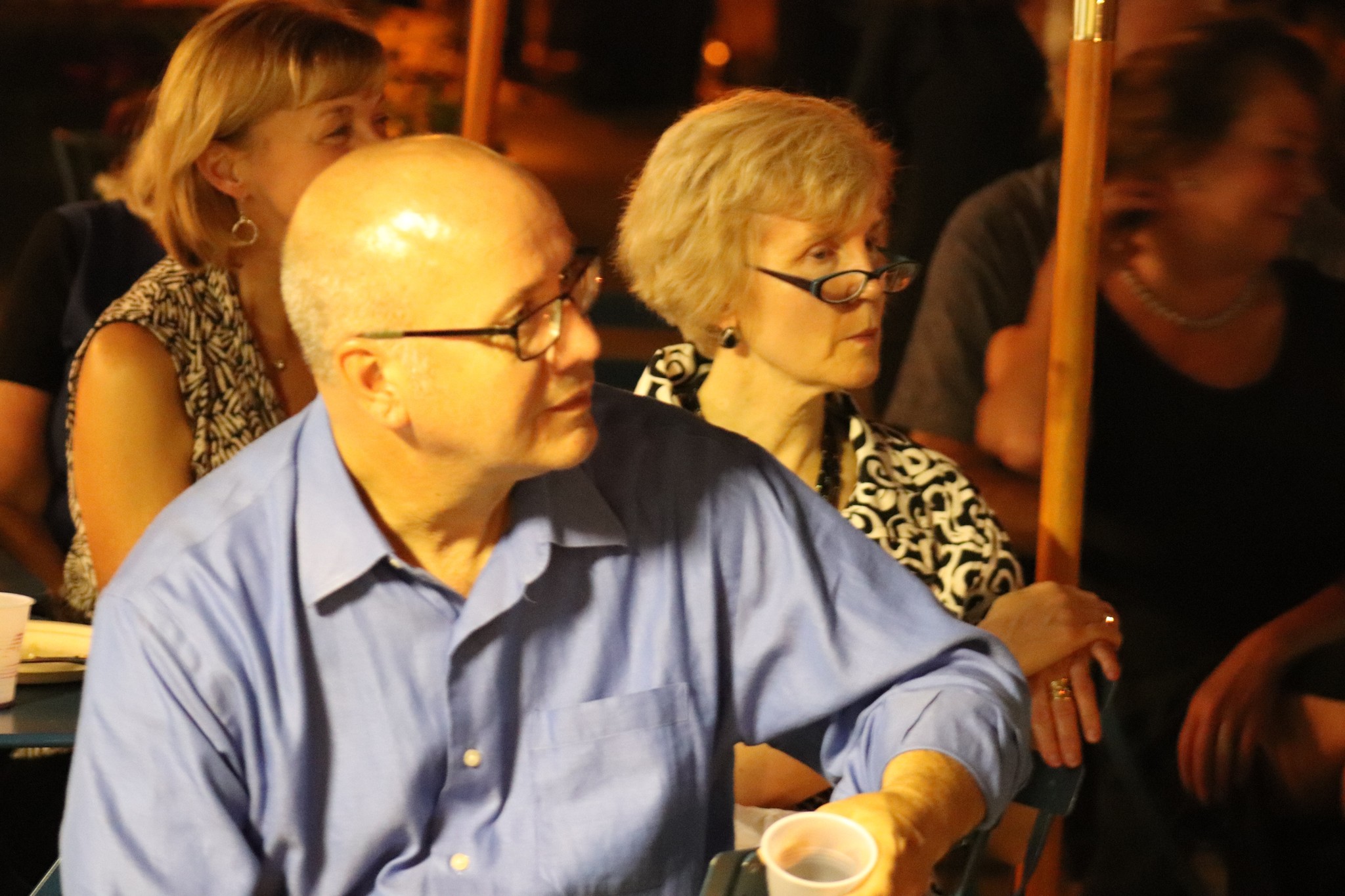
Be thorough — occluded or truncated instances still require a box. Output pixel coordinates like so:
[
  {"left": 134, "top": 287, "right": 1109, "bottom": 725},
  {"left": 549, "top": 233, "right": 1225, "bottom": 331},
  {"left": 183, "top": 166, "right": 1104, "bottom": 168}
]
[
  {"left": 740, "top": 242, "right": 923, "bottom": 305},
  {"left": 347, "top": 245, "right": 605, "bottom": 363}
]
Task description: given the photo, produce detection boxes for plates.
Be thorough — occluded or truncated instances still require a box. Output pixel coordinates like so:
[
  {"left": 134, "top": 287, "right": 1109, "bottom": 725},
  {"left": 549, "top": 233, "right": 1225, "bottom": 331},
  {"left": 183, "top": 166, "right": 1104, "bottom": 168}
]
[{"left": 17, "top": 619, "right": 96, "bottom": 684}]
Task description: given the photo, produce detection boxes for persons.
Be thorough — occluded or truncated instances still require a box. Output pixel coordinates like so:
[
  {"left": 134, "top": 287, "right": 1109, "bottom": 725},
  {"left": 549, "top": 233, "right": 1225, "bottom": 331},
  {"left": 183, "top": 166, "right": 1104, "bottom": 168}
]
[{"left": 0, "top": 0, "right": 1345, "bottom": 896}]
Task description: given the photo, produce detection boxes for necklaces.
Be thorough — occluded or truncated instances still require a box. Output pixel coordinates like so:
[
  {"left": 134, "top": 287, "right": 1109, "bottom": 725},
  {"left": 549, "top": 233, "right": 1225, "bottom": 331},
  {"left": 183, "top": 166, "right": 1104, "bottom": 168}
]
[
  {"left": 680, "top": 371, "right": 845, "bottom": 507},
  {"left": 1120, "top": 264, "right": 1262, "bottom": 331},
  {"left": 270, "top": 339, "right": 299, "bottom": 372}
]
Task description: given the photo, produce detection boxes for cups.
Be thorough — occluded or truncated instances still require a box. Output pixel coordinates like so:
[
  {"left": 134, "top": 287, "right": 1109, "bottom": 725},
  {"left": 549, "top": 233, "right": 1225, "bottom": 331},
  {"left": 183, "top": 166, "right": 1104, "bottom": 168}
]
[
  {"left": 0, "top": 592, "right": 36, "bottom": 709},
  {"left": 760, "top": 811, "right": 879, "bottom": 896}
]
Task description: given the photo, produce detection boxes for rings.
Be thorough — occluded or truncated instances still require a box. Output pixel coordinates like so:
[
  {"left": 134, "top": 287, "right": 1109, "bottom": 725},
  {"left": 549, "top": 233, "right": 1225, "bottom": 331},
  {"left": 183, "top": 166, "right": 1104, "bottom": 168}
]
[
  {"left": 1102, "top": 612, "right": 1116, "bottom": 626},
  {"left": 1050, "top": 677, "right": 1074, "bottom": 700}
]
